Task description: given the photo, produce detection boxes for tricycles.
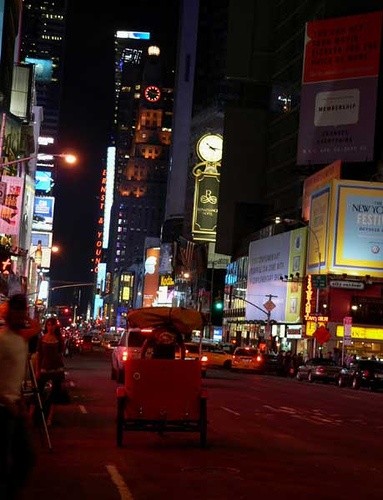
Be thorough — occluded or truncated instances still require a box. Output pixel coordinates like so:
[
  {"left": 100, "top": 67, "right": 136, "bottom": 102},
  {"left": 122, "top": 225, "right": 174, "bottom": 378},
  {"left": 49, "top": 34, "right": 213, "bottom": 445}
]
[{"left": 115, "top": 305, "right": 210, "bottom": 448}]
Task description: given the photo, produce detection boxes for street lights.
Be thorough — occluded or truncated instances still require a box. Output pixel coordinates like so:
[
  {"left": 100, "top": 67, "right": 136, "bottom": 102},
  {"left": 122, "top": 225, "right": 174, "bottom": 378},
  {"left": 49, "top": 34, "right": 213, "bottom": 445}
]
[{"left": 272, "top": 217, "right": 321, "bottom": 361}]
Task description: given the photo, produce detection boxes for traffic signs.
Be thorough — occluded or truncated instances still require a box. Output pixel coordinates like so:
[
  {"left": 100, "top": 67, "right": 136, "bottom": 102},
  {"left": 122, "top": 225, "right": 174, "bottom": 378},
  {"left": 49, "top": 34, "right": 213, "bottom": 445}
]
[{"left": 329, "top": 280, "right": 364, "bottom": 290}]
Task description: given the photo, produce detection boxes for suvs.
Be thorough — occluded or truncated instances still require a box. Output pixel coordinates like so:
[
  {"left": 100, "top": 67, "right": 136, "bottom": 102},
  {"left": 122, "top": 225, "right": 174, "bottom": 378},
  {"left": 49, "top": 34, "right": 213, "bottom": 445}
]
[{"left": 109, "top": 329, "right": 155, "bottom": 384}]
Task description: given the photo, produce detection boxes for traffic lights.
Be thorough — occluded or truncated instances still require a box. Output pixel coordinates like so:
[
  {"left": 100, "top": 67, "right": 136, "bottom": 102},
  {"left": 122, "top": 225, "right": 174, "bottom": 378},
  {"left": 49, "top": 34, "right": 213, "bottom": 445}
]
[{"left": 214, "top": 296, "right": 222, "bottom": 311}]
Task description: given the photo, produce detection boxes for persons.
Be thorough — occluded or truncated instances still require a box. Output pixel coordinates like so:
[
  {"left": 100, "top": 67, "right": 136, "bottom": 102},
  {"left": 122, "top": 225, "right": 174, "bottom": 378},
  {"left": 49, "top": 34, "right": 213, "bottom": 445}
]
[
  {"left": 141, "top": 325, "right": 186, "bottom": 360},
  {"left": 274, "top": 350, "right": 305, "bottom": 377},
  {"left": 329, "top": 347, "right": 376, "bottom": 370},
  {"left": 0, "top": 294, "right": 75, "bottom": 500}
]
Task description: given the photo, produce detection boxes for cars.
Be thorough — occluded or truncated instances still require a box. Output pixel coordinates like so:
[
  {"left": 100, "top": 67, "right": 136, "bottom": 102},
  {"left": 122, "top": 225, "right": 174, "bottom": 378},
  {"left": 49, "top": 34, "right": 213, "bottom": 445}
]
[
  {"left": 230, "top": 347, "right": 264, "bottom": 374},
  {"left": 175, "top": 342, "right": 232, "bottom": 371},
  {"left": 74, "top": 336, "right": 118, "bottom": 352},
  {"left": 295, "top": 358, "right": 342, "bottom": 384},
  {"left": 335, "top": 359, "right": 383, "bottom": 391}
]
